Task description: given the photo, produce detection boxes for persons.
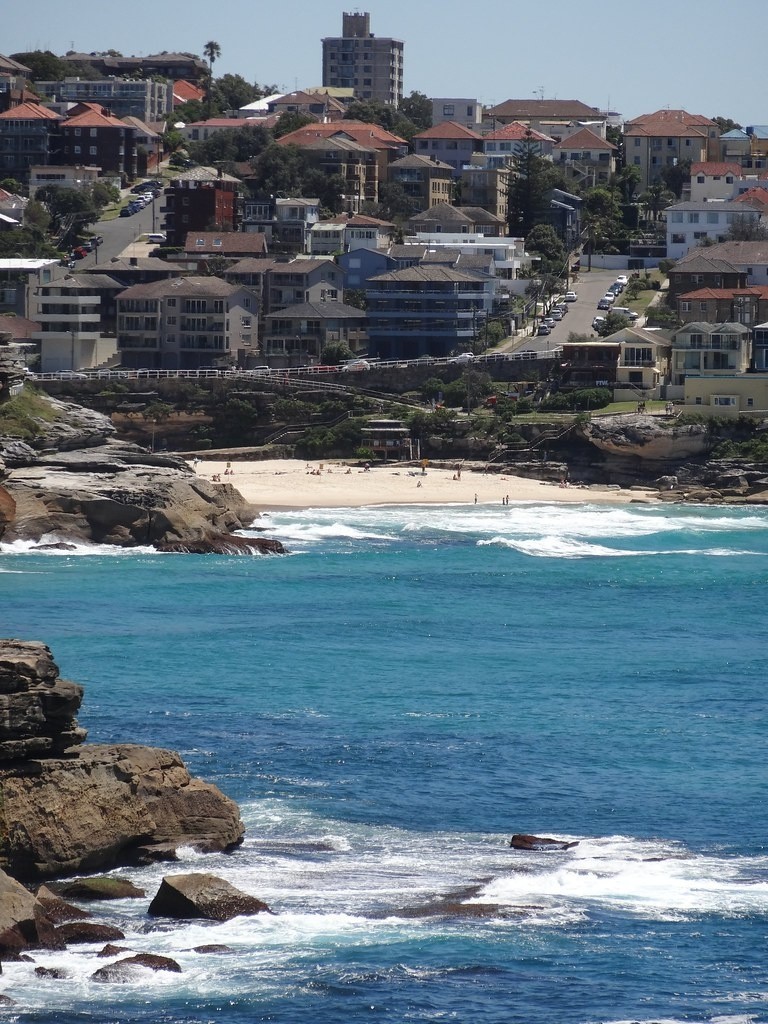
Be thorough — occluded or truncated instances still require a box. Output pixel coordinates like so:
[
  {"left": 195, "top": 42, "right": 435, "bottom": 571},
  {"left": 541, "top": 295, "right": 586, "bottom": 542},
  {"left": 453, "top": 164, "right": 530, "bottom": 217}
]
[
  {"left": 417, "top": 481, "right": 422, "bottom": 487},
  {"left": 213, "top": 474, "right": 220, "bottom": 482},
  {"left": 475, "top": 493, "right": 478, "bottom": 504},
  {"left": 503, "top": 495, "right": 509, "bottom": 504},
  {"left": 637, "top": 401, "right": 648, "bottom": 413},
  {"left": 559, "top": 480, "right": 569, "bottom": 488},
  {"left": 224, "top": 468, "right": 234, "bottom": 475},
  {"left": 453, "top": 468, "right": 461, "bottom": 480},
  {"left": 193, "top": 460, "right": 199, "bottom": 468},
  {"left": 344, "top": 467, "right": 351, "bottom": 474},
  {"left": 421, "top": 461, "right": 426, "bottom": 473},
  {"left": 328, "top": 468, "right": 332, "bottom": 473},
  {"left": 306, "top": 463, "right": 321, "bottom": 475},
  {"left": 665, "top": 403, "right": 673, "bottom": 412}
]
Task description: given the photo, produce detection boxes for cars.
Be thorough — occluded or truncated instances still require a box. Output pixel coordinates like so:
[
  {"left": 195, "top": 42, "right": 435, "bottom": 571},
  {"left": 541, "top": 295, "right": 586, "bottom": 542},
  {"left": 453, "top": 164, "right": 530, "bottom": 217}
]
[
  {"left": 251, "top": 366, "right": 270, "bottom": 376},
  {"left": 463, "top": 352, "right": 476, "bottom": 362},
  {"left": 62, "top": 236, "right": 104, "bottom": 268},
  {"left": 343, "top": 362, "right": 370, "bottom": 373},
  {"left": 512, "top": 349, "right": 537, "bottom": 359},
  {"left": 96, "top": 369, "right": 127, "bottom": 379},
  {"left": 480, "top": 353, "right": 509, "bottom": 361},
  {"left": 592, "top": 274, "right": 629, "bottom": 330},
  {"left": 120, "top": 180, "right": 164, "bottom": 217},
  {"left": 407, "top": 357, "right": 436, "bottom": 367},
  {"left": 538, "top": 303, "right": 569, "bottom": 336},
  {"left": 565, "top": 292, "right": 578, "bottom": 302},
  {"left": 56, "top": 370, "right": 88, "bottom": 379},
  {"left": 24, "top": 372, "right": 37, "bottom": 381},
  {"left": 447, "top": 354, "right": 475, "bottom": 366}
]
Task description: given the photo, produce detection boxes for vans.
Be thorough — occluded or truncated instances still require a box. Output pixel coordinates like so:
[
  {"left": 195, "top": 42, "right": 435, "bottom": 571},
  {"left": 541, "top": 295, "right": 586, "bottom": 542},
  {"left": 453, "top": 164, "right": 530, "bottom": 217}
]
[{"left": 608, "top": 307, "right": 638, "bottom": 320}]
